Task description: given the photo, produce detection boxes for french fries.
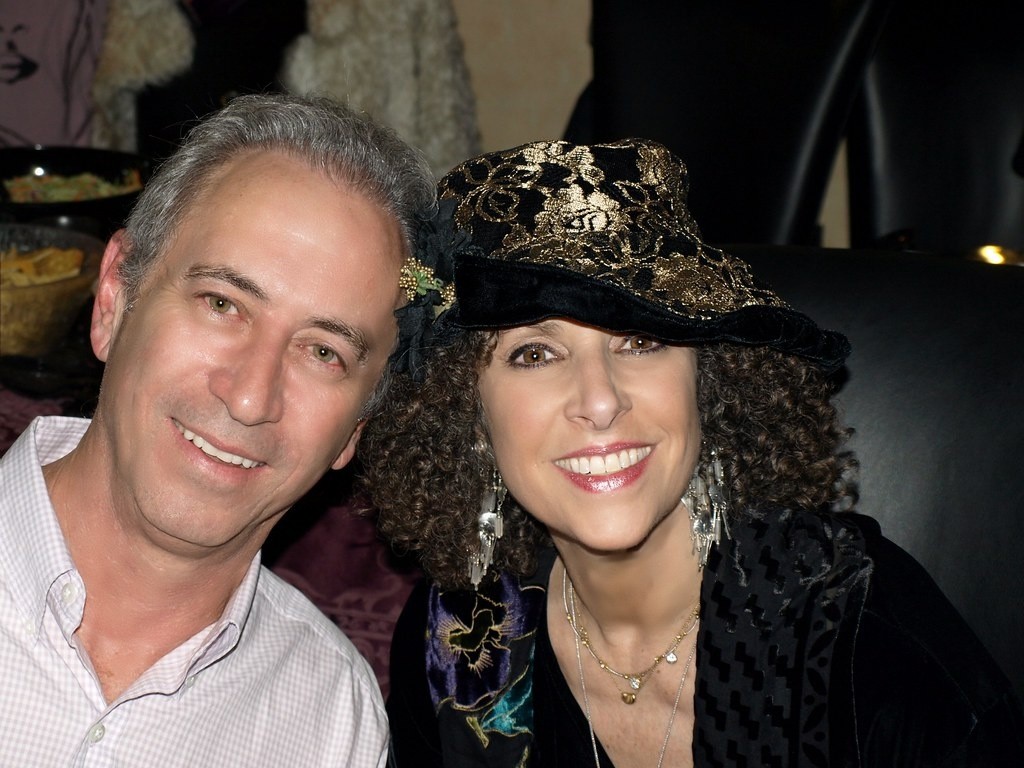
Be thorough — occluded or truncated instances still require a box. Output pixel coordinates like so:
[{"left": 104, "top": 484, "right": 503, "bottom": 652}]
[{"left": 0, "top": 243, "right": 83, "bottom": 289}]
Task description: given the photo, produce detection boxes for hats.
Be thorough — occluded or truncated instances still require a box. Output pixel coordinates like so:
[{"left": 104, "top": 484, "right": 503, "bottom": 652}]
[{"left": 393, "top": 142, "right": 852, "bottom": 398}]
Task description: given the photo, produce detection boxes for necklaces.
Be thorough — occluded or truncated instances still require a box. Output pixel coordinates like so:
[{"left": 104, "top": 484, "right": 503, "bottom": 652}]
[{"left": 559, "top": 553, "right": 702, "bottom": 767}]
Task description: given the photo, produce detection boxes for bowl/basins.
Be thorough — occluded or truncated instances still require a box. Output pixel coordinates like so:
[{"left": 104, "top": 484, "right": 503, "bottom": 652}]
[
  {"left": 0, "top": 146, "right": 160, "bottom": 239},
  {"left": 0, "top": 226, "right": 106, "bottom": 357}
]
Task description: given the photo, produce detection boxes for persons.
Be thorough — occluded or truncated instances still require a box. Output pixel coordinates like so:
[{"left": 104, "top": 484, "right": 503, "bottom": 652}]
[
  {"left": 354, "top": 133, "right": 1024, "bottom": 768},
  {"left": 1, "top": 91, "right": 438, "bottom": 767}
]
